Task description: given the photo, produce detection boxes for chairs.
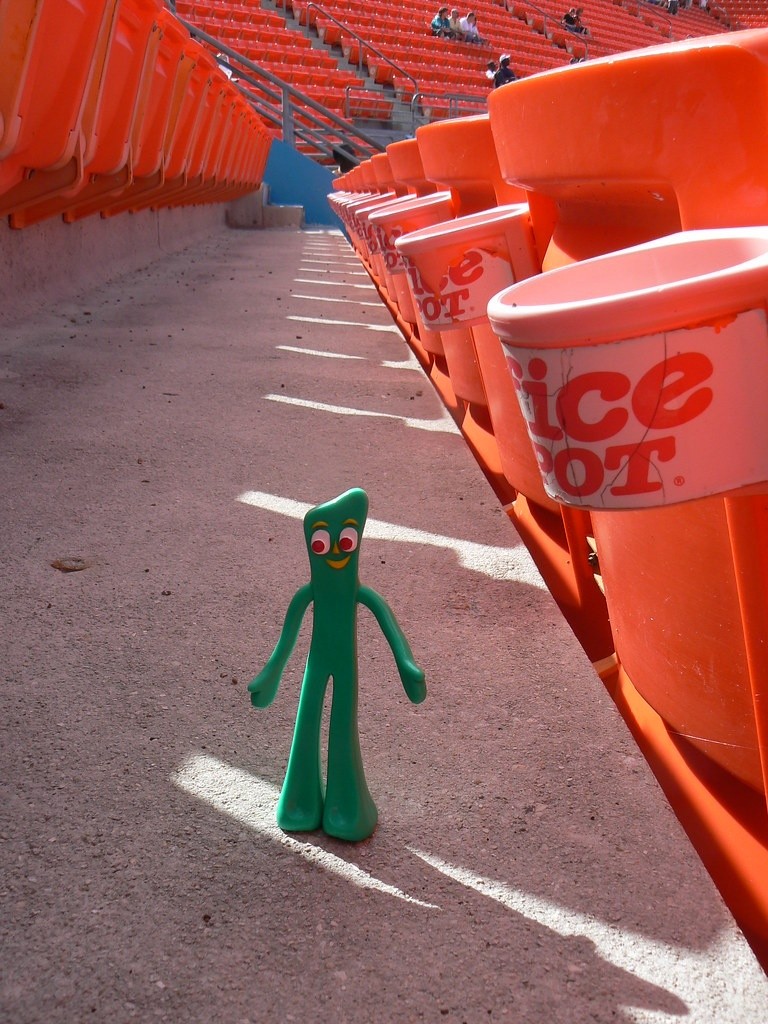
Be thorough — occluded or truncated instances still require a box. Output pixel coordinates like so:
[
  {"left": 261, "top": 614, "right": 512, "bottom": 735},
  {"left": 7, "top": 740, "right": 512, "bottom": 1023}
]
[{"left": 0, "top": 0, "right": 768, "bottom": 979}]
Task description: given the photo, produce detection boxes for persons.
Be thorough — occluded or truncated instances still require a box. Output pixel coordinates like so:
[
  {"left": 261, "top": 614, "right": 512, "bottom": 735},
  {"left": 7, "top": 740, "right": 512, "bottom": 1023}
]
[
  {"left": 560, "top": 7, "right": 588, "bottom": 35},
  {"left": 486, "top": 54, "right": 515, "bottom": 88},
  {"left": 569, "top": 57, "right": 585, "bottom": 64},
  {"left": 331, "top": 145, "right": 360, "bottom": 177},
  {"left": 190, "top": 32, "right": 201, "bottom": 44},
  {"left": 644, "top": 0, "right": 711, "bottom": 15},
  {"left": 217, "top": 53, "right": 240, "bottom": 83},
  {"left": 431, "top": 7, "right": 488, "bottom": 44}
]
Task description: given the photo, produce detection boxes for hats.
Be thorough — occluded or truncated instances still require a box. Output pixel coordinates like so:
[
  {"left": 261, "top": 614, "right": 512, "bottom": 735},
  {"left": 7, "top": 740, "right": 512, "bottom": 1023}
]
[
  {"left": 499, "top": 53, "right": 511, "bottom": 63},
  {"left": 486, "top": 61, "right": 499, "bottom": 68}
]
